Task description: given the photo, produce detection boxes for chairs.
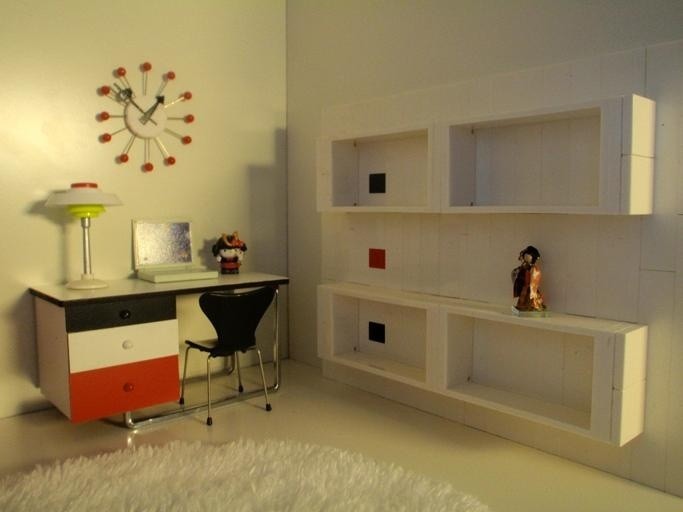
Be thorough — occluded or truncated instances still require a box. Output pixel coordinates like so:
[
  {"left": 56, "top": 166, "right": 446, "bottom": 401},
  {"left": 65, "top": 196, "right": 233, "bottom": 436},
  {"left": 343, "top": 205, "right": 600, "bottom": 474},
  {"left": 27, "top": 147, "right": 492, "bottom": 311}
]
[{"left": 179, "top": 282, "right": 279, "bottom": 426}]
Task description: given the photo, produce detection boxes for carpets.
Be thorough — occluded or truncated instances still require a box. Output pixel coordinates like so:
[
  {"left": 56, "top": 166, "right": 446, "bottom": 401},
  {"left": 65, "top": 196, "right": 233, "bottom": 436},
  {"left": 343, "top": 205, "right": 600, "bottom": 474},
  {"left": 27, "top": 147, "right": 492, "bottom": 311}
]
[{"left": 1, "top": 442, "right": 492, "bottom": 512}]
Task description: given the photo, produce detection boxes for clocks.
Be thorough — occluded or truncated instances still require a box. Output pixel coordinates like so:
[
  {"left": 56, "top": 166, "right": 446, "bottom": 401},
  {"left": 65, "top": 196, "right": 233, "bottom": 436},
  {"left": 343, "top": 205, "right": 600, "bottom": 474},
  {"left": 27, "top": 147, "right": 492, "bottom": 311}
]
[{"left": 99, "top": 61, "right": 196, "bottom": 172}]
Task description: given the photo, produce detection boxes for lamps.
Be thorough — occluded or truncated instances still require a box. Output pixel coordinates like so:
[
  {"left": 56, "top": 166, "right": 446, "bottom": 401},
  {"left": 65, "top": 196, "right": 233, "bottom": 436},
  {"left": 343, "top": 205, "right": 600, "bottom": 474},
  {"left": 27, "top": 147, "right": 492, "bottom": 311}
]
[{"left": 45, "top": 182, "right": 124, "bottom": 291}]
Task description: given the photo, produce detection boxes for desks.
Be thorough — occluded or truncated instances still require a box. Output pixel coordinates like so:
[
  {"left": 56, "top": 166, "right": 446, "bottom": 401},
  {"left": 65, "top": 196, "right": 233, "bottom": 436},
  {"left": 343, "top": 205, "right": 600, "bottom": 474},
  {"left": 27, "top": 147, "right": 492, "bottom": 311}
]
[{"left": 29, "top": 271, "right": 290, "bottom": 429}]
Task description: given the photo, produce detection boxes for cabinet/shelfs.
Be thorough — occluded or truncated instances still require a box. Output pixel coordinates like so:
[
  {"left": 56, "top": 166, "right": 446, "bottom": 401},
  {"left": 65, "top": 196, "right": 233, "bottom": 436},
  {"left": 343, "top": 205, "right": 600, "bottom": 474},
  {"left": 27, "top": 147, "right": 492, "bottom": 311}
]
[
  {"left": 312, "top": 94, "right": 660, "bottom": 220},
  {"left": 313, "top": 282, "right": 648, "bottom": 447}
]
